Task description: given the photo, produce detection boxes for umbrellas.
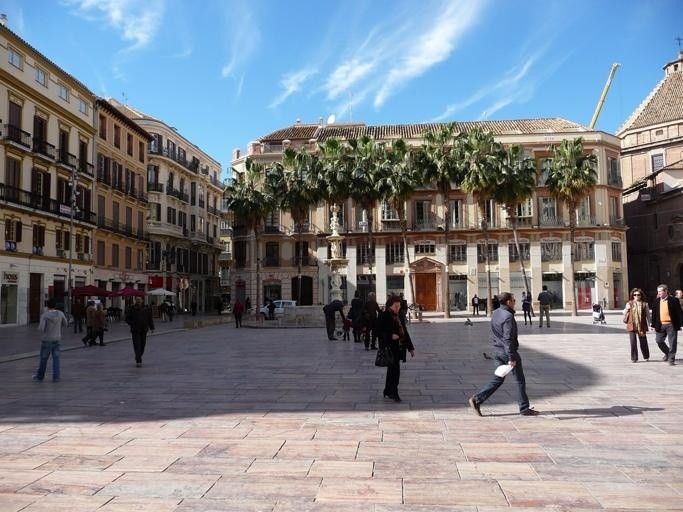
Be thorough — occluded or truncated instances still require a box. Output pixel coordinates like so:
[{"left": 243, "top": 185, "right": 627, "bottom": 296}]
[
  {"left": 110, "top": 287, "right": 144, "bottom": 296},
  {"left": 146, "top": 288, "right": 176, "bottom": 306},
  {"left": 71, "top": 284, "right": 112, "bottom": 297}
]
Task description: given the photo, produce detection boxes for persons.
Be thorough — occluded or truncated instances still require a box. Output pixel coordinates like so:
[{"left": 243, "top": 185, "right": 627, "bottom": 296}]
[
  {"left": 72, "top": 295, "right": 177, "bottom": 369},
  {"left": 191, "top": 299, "right": 197, "bottom": 317},
  {"left": 233, "top": 299, "right": 244, "bottom": 328},
  {"left": 323, "top": 289, "right": 415, "bottom": 402},
  {"left": 651, "top": 284, "right": 683, "bottom": 366},
  {"left": 245, "top": 297, "right": 251, "bottom": 309},
  {"left": 267, "top": 299, "right": 276, "bottom": 320},
  {"left": 622, "top": 288, "right": 652, "bottom": 363},
  {"left": 674, "top": 289, "right": 683, "bottom": 312},
  {"left": 31, "top": 299, "right": 69, "bottom": 382},
  {"left": 468, "top": 285, "right": 553, "bottom": 417},
  {"left": 217, "top": 301, "right": 223, "bottom": 315}
]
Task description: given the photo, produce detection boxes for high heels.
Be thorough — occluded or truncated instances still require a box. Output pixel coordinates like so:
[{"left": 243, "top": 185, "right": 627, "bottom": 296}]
[{"left": 383, "top": 389, "right": 401, "bottom": 403}]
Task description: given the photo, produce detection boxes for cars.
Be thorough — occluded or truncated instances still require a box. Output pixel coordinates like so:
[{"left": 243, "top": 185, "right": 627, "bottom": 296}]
[{"left": 256, "top": 300, "right": 297, "bottom": 319}]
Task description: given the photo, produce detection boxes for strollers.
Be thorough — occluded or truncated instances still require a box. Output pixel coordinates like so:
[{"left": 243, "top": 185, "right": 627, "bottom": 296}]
[{"left": 591, "top": 303, "right": 605, "bottom": 326}]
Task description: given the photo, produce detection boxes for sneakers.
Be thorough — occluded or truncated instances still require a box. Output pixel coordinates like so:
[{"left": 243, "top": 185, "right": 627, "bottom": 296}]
[
  {"left": 645, "top": 359, "right": 649, "bottom": 361},
  {"left": 632, "top": 359, "right": 638, "bottom": 363},
  {"left": 328, "top": 338, "right": 362, "bottom": 342},
  {"left": 520, "top": 409, "right": 539, "bottom": 416},
  {"left": 32, "top": 375, "right": 42, "bottom": 382},
  {"left": 663, "top": 355, "right": 669, "bottom": 361},
  {"left": 82, "top": 337, "right": 106, "bottom": 346},
  {"left": 137, "top": 362, "right": 143, "bottom": 367},
  {"left": 468, "top": 396, "right": 482, "bottom": 417},
  {"left": 371, "top": 346, "right": 378, "bottom": 350},
  {"left": 669, "top": 362, "right": 674, "bottom": 366}
]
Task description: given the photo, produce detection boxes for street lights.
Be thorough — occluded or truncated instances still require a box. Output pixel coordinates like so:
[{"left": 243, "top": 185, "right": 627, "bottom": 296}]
[{"left": 65, "top": 161, "right": 81, "bottom": 327}]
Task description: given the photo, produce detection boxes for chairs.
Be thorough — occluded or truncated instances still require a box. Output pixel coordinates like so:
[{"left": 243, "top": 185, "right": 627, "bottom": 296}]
[{"left": 99, "top": 303, "right": 160, "bottom": 327}]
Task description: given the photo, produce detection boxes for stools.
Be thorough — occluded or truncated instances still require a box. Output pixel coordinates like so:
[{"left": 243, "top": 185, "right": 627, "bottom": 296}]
[
  {"left": 255, "top": 316, "right": 263, "bottom": 325},
  {"left": 294, "top": 316, "right": 304, "bottom": 326}
]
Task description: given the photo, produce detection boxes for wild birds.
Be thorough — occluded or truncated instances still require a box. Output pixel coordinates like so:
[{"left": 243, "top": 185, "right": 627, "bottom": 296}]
[{"left": 484, "top": 352, "right": 494, "bottom": 362}]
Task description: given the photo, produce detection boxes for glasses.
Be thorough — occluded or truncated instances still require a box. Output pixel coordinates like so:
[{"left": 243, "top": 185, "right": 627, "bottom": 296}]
[{"left": 633, "top": 294, "right": 641, "bottom": 296}]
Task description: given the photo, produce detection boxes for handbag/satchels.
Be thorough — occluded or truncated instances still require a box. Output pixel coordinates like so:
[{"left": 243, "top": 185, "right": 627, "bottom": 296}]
[
  {"left": 623, "top": 311, "right": 630, "bottom": 323},
  {"left": 375, "top": 345, "right": 394, "bottom": 368}
]
